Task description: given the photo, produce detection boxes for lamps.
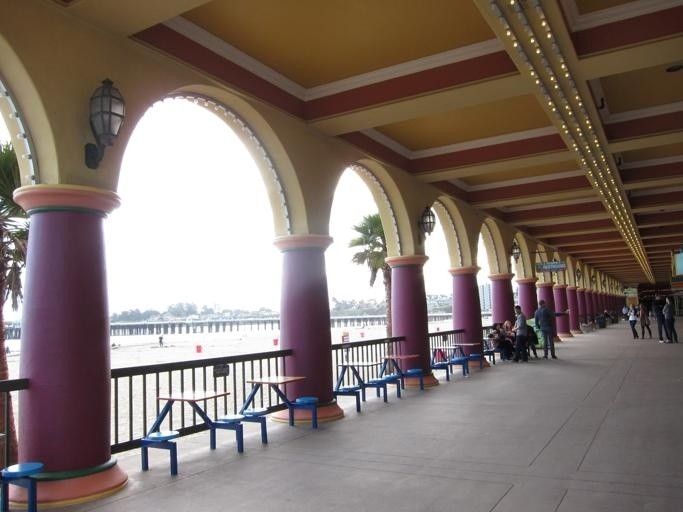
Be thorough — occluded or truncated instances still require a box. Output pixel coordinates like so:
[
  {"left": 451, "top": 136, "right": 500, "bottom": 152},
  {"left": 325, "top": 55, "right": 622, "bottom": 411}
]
[
  {"left": 591, "top": 275, "right": 624, "bottom": 293},
  {"left": 83, "top": 79, "right": 125, "bottom": 169},
  {"left": 417, "top": 205, "right": 435, "bottom": 236},
  {"left": 510, "top": 241, "right": 521, "bottom": 263},
  {"left": 575, "top": 267, "right": 582, "bottom": 280}
]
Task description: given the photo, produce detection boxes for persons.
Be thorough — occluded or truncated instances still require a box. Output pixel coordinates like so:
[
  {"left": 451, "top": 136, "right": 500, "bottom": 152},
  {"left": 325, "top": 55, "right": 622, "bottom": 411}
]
[
  {"left": 627, "top": 304, "right": 639, "bottom": 339},
  {"left": 652, "top": 292, "right": 671, "bottom": 343},
  {"left": 489, "top": 321, "right": 539, "bottom": 361},
  {"left": 159, "top": 335, "right": 163, "bottom": 347},
  {"left": 596, "top": 303, "right": 652, "bottom": 328},
  {"left": 535, "top": 299, "right": 570, "bottom": 359},
  {"left": 663, "top": 296, "right": 678, "bottom": 344},
  {"left": 639, "top": 304, "right": 652, "bottom": 339},
  {"left": 511, "top": 305, "right": 529, "bottom": 362}
]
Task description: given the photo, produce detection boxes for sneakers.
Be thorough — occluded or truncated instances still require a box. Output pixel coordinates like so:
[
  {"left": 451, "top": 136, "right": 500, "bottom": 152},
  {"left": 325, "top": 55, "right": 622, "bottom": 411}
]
[
  {"left": 498, "top": 355, "right": 558, "bottom": 364},
  {"left": 634, "top": 336, "right": 679, "bottom": 344}
]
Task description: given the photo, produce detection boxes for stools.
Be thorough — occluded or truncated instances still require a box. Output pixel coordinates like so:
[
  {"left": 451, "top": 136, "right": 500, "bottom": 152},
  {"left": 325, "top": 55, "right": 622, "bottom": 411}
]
[
  {"left": 450, "top": 358, "right": 466, "bottom": 375},
  {"left": 0, "top": 462, "right": 45, "bottom": 512},
  {"left": 454, "top": 356, "right": 469, "bottom": 373},
  {"left": 400, "top": 368, "right": 424, "bottom": 390},
  {"left": 333, "top": 385, "right": 361, "bottom": 412},
  {"left": 383, "top": 374, "right": 400, "bottom": 398},
  {"left": 210, "top": 414, "right": 244, "bottom": 452},
  {"left": 430, "top": 361, "right": 449, "bottom": 381},
  {"left": 362, "top": 378, "right": 387, "bottom": 402},
  {"left": 236, "top": 407, "right": 268, "bottom": 443},
  {"left": 466, "top": 352, "right": 483, "bottom": 369},
  {"left": 141, "top": 431, "right": 180, "bottom": 475},
  {"left": 288, "top": 396, "right": 319, "bottom": 429}
]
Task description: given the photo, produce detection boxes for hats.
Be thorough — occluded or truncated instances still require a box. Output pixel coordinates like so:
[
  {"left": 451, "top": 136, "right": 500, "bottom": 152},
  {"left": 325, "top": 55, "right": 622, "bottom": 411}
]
[{"left": 629, "top": 304, "right": 635, "bottom": 307}]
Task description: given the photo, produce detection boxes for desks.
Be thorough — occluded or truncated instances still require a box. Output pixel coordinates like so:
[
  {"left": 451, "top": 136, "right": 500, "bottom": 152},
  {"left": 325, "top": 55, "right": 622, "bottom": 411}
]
[
  {"left": 429, "top": 347, "right": 459, "bottom": 365},
  {"left": 147, "top": 391, "right": 230, "bottom": 437},
  {"left": 335, "top": 361, "right": 382, "bottom": 390},
  {"left": 239, "top": 376, "right": 309, "bottom": 415},
  {"left": 451, "top": 343, "right": 480, "bottom": 358},
  {"left": 379, "top": 355, "right": 420, "bottom": 378}
]
